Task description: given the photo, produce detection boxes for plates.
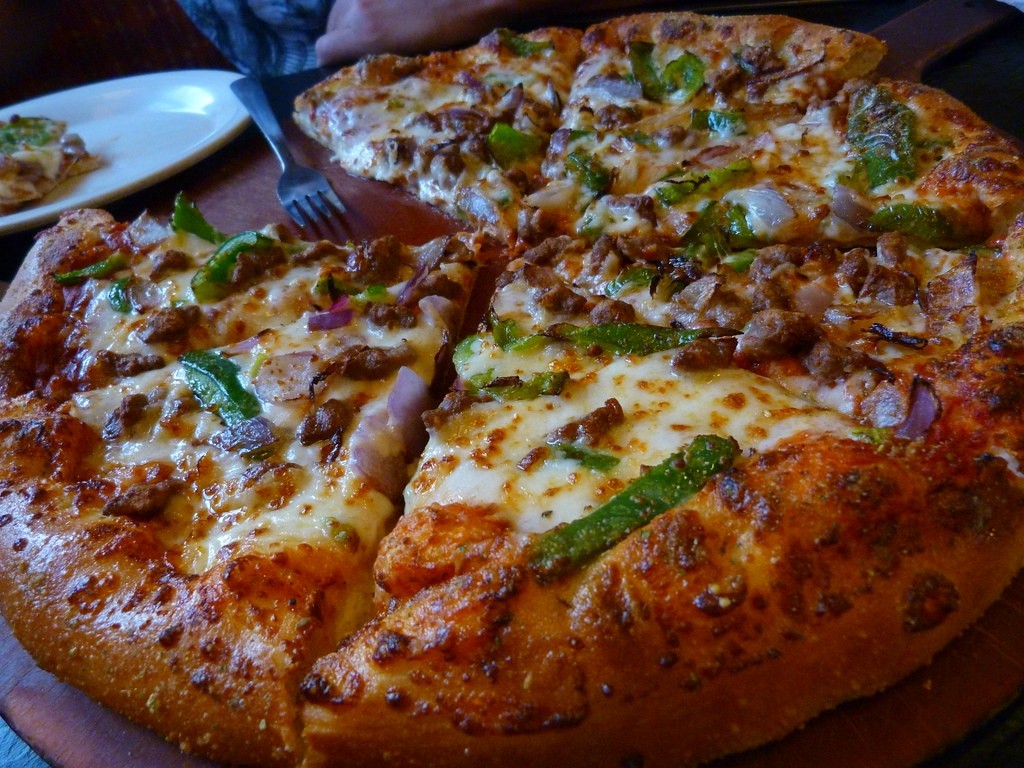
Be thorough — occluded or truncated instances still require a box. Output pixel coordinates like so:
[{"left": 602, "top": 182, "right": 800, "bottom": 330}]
[{"left": 0, "top": 69, "right": 254, "bottom": 237}]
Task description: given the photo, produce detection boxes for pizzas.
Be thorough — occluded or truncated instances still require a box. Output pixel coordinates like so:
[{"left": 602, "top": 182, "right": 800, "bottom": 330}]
[{"left": 0, "top": 11, "right": 1024, "bottom": 768}]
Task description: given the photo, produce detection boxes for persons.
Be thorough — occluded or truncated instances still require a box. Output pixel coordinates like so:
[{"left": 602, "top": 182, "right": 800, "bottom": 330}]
[{"left": 176, "top": 1, "right": 551, "bottom": 78}]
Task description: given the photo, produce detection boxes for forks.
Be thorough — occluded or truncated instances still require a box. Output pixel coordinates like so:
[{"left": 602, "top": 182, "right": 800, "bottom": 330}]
[{"left": 230, "top": 78, "right": 346, "bottom": 227}]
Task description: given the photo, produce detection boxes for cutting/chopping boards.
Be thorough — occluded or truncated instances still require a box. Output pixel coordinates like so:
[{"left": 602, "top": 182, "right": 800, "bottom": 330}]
[{"left": 0, "top": 0, "right": 1024, "bottom": 768}]
